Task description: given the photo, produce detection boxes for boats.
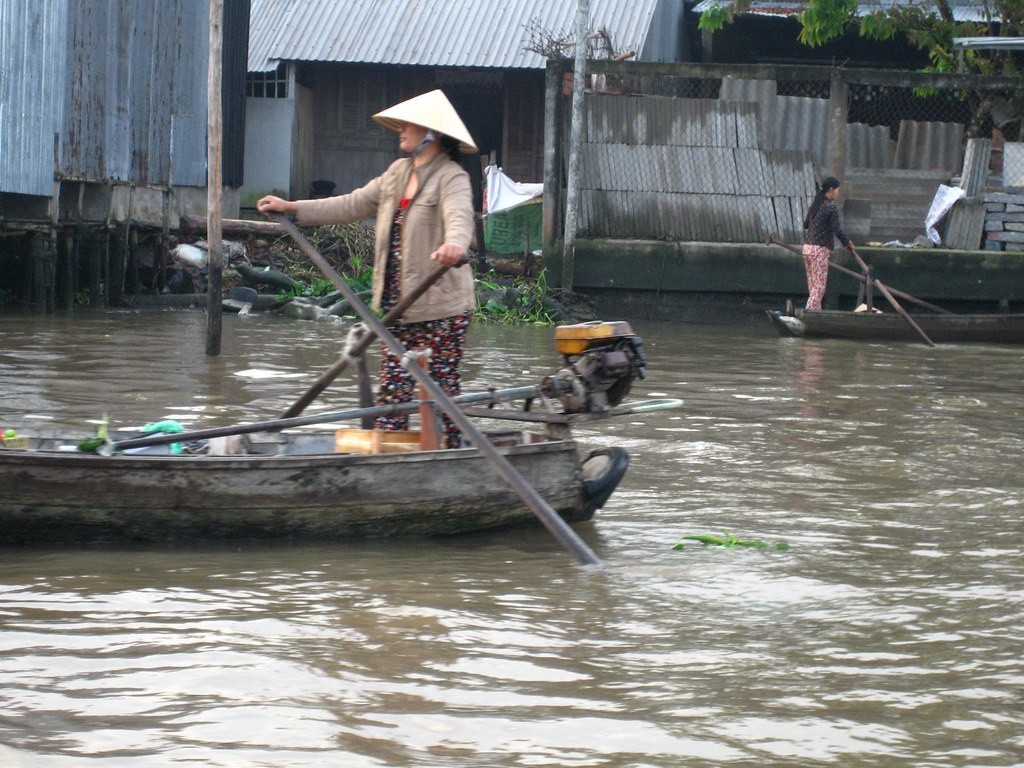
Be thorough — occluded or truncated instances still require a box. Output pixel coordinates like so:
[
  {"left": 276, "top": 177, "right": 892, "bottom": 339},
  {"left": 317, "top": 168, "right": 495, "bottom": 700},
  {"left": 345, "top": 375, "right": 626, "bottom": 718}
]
[
  {"left": 0, "top": 425, "right": 628, "bottom": 541},
  {"left": 765, "top": 299, "right": 1024, "bottom": 341}
]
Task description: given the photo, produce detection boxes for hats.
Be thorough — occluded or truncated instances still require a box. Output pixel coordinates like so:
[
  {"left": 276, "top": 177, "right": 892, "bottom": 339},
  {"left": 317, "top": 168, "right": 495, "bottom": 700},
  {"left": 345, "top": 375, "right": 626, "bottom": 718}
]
[{"left": 371, "top": 89, "right": 478, "bottom": 153}]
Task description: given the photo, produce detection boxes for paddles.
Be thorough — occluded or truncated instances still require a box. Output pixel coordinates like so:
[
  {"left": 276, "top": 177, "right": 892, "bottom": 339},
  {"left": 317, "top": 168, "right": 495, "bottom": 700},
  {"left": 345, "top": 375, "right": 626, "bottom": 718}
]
[
  {"left": 764, "top": 231, "right": 951, "bottom": 315},
  {"left": 260, "top": 197, "right": 605, "bottom": 565},
  {"left": 848, "top": 248, "right": 936, "bottom": 347},
  {"left": 266, "top": 253, "right": 471, "bottom": 432}
]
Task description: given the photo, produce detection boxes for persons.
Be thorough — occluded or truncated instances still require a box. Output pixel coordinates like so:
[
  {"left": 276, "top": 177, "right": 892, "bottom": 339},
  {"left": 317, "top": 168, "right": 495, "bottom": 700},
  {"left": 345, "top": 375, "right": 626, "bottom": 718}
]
[
  {"left": 801, "top": 176, "right": 855, "bottom": 309},
  {"left": 257, "top": 90, "right": 479, "bottom": 448}
]
